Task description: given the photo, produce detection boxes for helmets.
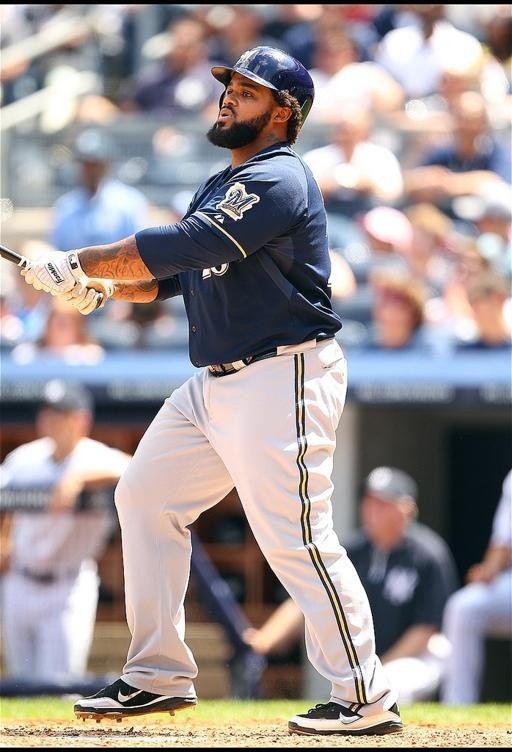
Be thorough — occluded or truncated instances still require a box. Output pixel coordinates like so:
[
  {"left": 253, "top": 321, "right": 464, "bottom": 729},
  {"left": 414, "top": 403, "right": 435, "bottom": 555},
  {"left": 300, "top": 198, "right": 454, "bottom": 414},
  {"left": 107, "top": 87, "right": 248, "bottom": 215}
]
[{"left": 211, "top": 44, "right": 316, "bottom": 139}]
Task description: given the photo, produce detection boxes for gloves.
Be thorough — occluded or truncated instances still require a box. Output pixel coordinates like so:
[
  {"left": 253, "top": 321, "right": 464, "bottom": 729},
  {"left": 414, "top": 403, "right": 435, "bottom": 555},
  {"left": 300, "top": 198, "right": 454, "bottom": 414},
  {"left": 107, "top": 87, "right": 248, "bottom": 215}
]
[
  {"left": 20, "top": 249, "right": 86, "bottom": 297},
  {"left": 60, "top": 277, "right": 114, "bottom": 316}
]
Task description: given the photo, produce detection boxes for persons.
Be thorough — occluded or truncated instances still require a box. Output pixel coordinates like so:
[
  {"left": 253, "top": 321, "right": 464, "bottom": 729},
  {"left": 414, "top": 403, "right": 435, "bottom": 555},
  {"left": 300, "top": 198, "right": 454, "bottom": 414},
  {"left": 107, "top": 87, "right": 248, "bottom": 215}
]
[{"left": 18, "top": 43, "right": 413, "bottom": 736}]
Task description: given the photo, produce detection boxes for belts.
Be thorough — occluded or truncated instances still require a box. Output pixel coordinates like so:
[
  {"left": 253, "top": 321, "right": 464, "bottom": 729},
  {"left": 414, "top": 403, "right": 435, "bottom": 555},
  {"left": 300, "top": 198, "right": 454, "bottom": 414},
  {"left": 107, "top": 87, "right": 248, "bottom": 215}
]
[{"left": 207, "top": 332, "right": 335, "bottom": 378}]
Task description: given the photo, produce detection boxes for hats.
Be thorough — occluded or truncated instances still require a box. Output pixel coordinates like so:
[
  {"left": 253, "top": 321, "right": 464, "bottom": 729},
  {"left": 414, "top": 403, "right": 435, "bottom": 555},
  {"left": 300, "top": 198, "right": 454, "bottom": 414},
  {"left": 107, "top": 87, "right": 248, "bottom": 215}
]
[
  {"left": 363, "top": 465, "right": 418, "bottom": 511},
  {"left": 34, "top": 376, "right": 94, "bottom": 412}
]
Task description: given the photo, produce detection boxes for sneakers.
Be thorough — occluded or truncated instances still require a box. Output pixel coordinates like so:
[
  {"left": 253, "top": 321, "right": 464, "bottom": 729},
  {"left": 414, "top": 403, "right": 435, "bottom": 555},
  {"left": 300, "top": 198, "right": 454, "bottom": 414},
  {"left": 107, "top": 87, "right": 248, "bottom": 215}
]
[
  {"left": 287, "top": 689, "right": 403, "bottom": 737},
  {"left": 73, "top": 671, "right": 197, "bottom": 724}
]
